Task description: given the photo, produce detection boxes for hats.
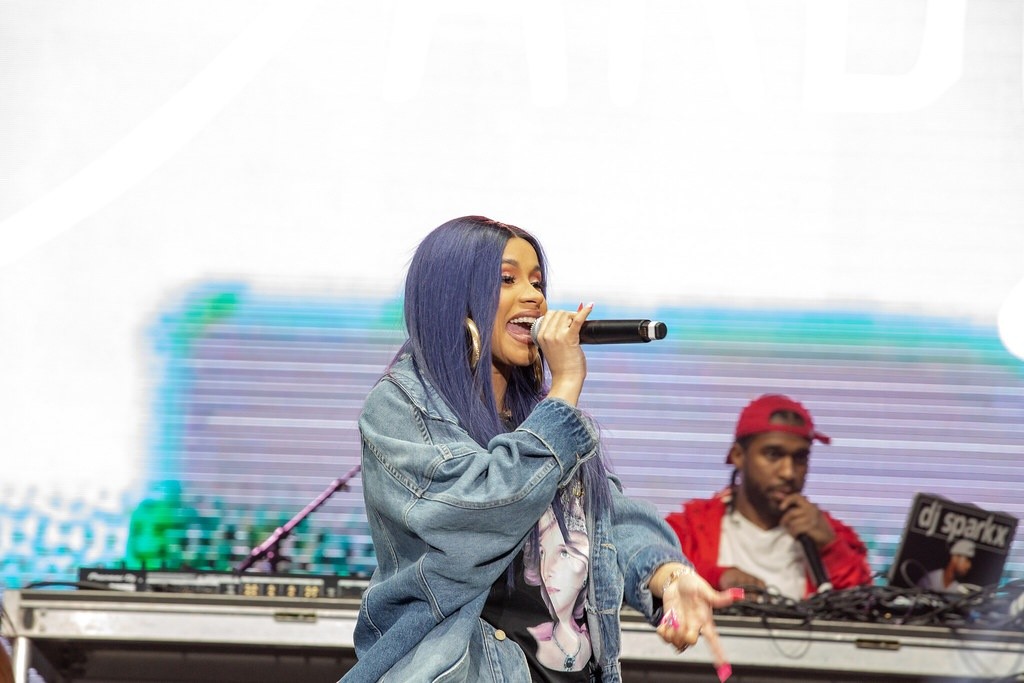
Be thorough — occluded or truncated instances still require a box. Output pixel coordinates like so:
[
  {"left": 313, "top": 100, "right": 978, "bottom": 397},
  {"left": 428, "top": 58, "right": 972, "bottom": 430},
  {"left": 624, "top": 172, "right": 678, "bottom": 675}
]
[
  {"left": 950, "top": 539, "right": 976, "bottom": 559},
  {"left": 725, "top": 394, "right": 831, "bottom": 465}
]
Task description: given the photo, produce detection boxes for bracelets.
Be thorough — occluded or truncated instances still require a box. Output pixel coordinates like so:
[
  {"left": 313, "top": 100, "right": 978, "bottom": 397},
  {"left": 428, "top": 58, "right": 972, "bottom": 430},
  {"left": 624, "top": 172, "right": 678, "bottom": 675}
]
[{"left": 661, "top": 567, "right": 693, "bottom": 591}]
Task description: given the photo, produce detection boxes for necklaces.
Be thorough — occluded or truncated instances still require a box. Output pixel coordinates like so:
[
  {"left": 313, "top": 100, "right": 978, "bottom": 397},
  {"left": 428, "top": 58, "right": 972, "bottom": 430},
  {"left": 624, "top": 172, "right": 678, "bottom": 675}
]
[{"left": 552, "top": 620, "right": 583, "bottom": 672}]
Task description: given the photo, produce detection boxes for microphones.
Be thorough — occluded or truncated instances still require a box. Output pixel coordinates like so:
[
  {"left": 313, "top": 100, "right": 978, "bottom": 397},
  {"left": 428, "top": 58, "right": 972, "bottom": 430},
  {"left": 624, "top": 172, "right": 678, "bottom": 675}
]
[
  {"left": 530, "top": 316, "right": 667, "bottom": 349},
  {"left": 788, "top": 500, "right": 833, "bottom": 594}
]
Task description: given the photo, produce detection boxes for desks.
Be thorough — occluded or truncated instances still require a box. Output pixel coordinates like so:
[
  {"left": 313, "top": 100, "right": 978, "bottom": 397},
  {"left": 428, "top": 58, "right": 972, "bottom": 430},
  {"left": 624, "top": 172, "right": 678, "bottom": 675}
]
[{"left": 7, "top": 591, "right": 1023, "bottom": 683}]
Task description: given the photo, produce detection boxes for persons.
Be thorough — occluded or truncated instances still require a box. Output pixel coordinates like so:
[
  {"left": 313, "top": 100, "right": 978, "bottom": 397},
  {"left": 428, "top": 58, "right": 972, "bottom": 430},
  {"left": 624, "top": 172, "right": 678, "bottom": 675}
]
[
  {"left": 665, "top": 395, "right": 873, "bottom": 603},
  {"left": 482, "top": 480, "right": 604, "bottom": 683},
  {"left": 917, "top": 540, "right": 977, "bottom": 595},
  {"left": 335, "top": 216, "right": 745, "bottom": 683}
]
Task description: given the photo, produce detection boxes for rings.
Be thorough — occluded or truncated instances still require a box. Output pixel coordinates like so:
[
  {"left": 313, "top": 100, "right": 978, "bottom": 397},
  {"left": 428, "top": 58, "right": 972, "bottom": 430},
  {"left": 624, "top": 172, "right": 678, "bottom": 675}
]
[{"left": 566, "top": 318, "right": 574, "bottom": 327}]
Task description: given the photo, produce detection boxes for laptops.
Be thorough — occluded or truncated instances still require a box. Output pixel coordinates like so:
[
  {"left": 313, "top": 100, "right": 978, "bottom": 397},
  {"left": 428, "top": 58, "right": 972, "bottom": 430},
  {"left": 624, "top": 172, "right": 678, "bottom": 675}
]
[{"left": 886, "top": 492, "right": 1017, "bottom": 594}]
